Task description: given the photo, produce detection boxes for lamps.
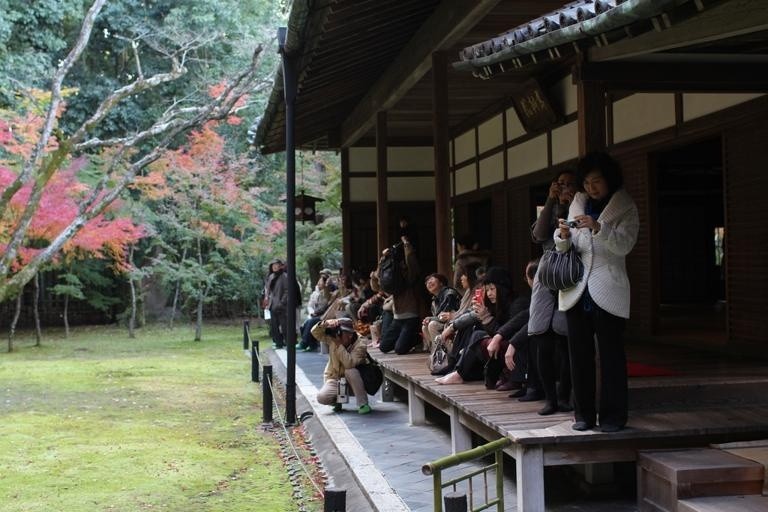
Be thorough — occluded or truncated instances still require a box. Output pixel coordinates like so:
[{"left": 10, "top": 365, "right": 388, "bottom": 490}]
[{"left": 280, "top": 147, "right": 328, "bottom": 226}]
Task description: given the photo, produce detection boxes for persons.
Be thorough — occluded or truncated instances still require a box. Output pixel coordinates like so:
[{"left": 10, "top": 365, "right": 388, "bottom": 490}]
[
  {"left": 526, "top": 151, "right": 640, "bottom": 431},
  {"left": 295, "top": 268, "right": 384, "bottom": 414},
  {"left": 371, "top": 219, "right": 546, "bottom": 402},
  {"left": 263, "top": 258, "right": 301, "bottom": 349}
]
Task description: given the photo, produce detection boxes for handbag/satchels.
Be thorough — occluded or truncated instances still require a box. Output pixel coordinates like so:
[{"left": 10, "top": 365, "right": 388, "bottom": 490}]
[
  {"left": 427, "top": 336, "right": 449, "bottom": 374},
  {"left": 376, "top": 239, "right": 409, "bottom": 295},
  {"left": 355, "top": 349, "right": 384, "bottom": 396},
  {"left": 537, "top": 235, "right": 584, "bottom": 289}
]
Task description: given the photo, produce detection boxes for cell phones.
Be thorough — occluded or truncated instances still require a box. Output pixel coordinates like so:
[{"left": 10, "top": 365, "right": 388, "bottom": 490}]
[{"left": 475, "top": 288, "right": 482, "bottom": 305}]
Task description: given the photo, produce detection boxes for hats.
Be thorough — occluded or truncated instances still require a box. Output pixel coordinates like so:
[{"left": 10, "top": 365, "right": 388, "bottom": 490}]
[
  {"left": 337, "top": 316, "right": 354, "bottom": 333},
  {"left": 319, "top": 268, "right": 332, "bottom": 277},
  {"left": 269, "top": 258, "right": 284, "bottom": 272}
]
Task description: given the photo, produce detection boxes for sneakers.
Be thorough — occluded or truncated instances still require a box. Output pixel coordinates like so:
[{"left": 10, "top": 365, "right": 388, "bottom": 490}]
[
  {"left": 332, "top": 402, "right": 342, "bottom": 412},
  {"left": 572, "top": 421, "right": 596, "bottom": 431},
  {"left": 296, "top": 343, "right": 312, "bottom": 352},
  {"left": 485, "top": 379, "right": 523, "bottom": 391},
  {"left": 600, "top": 423, "right": 623, "bottom": 432},
  {"left": 358, "top": 403, "right": 372, "bottom": 415},
  {"left": 509, "top": 385, "right": 546, "bottom": 402}
]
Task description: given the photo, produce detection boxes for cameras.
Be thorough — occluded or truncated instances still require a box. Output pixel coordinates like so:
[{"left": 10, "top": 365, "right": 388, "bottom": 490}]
[
  {"left": 563, "top": 219, "right": 580, "bottom": 228},
  {"left": 325, "top": 327, "right": 341, "bottom": 338}
]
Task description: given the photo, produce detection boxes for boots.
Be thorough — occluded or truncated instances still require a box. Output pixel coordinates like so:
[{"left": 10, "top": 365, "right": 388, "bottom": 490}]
[
  {"left": 537, "top": 382, "right": 558, "bottom": 415},
  {"left": 557, "top": 382, "right": 574, "bottom": 412}
]
